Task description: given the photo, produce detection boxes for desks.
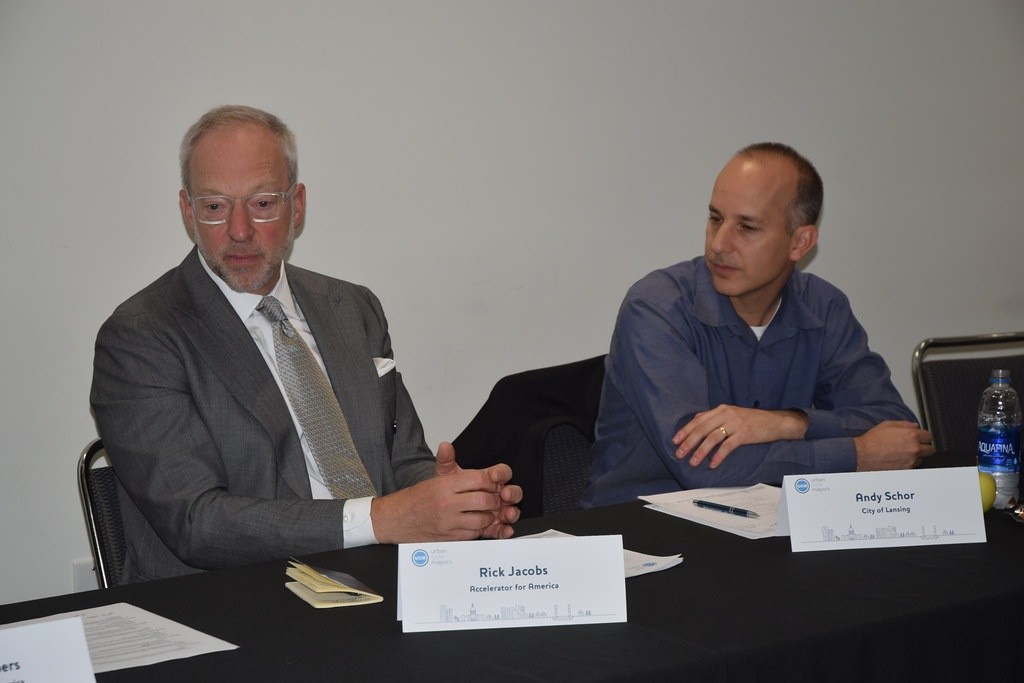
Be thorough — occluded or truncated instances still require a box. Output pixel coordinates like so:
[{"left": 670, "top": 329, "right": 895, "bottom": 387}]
[{"left": 0, "top": 453, "right": 1024, "bottom": 683}]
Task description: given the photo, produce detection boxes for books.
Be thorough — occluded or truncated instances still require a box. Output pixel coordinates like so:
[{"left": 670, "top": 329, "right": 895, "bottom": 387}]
[{"left": 284, "top": 555, "right": 383, "bottom": 609}]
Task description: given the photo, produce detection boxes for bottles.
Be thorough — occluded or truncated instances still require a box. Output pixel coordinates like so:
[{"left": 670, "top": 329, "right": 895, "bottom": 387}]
[{"left": 976, "top": 370, "right": 1022, "bottom": 510}]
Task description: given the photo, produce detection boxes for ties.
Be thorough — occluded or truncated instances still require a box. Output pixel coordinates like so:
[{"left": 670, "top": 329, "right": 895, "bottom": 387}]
[{"left": 257, "top": 297, "right": 379, "bottom": 499}]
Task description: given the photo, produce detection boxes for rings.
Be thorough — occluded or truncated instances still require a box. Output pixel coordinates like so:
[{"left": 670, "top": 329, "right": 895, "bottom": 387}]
[{"left": 718, "top": 425, "right": 729, "bottom": 437}]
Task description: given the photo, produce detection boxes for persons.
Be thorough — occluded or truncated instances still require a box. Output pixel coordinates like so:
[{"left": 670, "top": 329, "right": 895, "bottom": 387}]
[
  {"left": 90, "top": 103, "right": 522, "bottom": 585},
  {"left": 578, "top": 141, "right": 936, "bottom": 510}
]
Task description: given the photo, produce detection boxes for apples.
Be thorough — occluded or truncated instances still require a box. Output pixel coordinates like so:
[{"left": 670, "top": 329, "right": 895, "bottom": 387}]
[{"left": 979, "top": 471, "right": 997, "bottom": 514}]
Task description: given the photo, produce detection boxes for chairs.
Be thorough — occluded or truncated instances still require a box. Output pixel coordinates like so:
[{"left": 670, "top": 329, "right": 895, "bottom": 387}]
[
  {"left": 452, "top": 353, "right": 606, "bottom": 519},
  {"left": 77, "top": 436, "right": 125, "bottom": 589},
  {"left": 909, "top": 332, "right": 1024, "bottom": 454}
]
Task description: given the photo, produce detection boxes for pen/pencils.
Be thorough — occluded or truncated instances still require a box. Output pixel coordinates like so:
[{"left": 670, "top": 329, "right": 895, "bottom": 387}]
[{"left": 693, "top": 500, "right": 761, "bottom": 521}]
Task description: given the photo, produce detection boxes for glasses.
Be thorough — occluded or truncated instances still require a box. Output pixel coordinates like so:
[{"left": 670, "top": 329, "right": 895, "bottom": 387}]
[{"left": 185, "top": 186, "right": 294, "bottom": 225}]
